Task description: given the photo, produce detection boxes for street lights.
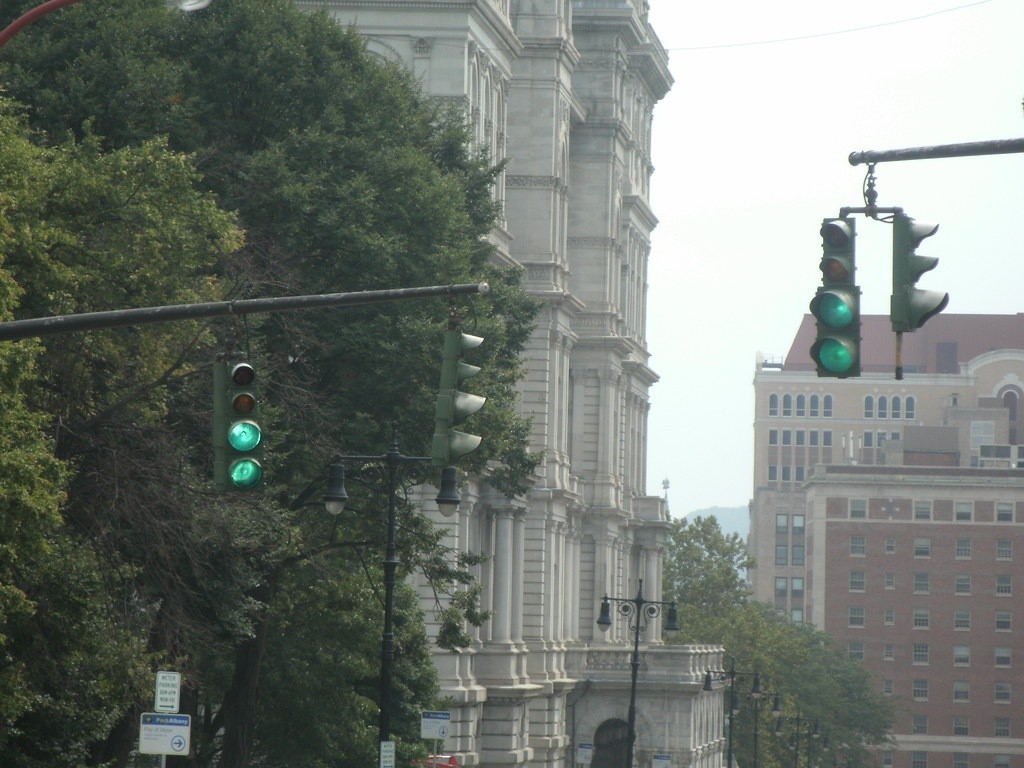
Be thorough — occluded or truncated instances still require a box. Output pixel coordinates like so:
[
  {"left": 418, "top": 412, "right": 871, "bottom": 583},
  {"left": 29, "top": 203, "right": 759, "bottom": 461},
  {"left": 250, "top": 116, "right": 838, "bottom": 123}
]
[
  {"left": 775, "top": 705, "right": 820, "bottom": 768},
  {"left": 594, "top": 578, "right": 682, "bottom": 768},
  {"left": 732, "top": 686, "right": 783, "bottom": 768},
  {"left": 323, "top": 428, "right": 462, "bottom": 767},
  {"left": 703, "top": 656, "right": 764, "bottom": 768}
]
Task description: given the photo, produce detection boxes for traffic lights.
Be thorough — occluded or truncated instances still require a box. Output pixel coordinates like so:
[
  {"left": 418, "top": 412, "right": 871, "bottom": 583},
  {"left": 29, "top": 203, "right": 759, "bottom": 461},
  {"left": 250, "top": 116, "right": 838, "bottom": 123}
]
[
  {"left": 809, "top": 218, "right": 863, "bottom": 379},
  {"left": 431, "top": 321, "right": 486, "bottom": 470},
  {"left": 889, "top": 211, "right": 949, "bottom": 332},
  {"left": 213, "top": 356, "right": 266, "bottom": 497}
]
